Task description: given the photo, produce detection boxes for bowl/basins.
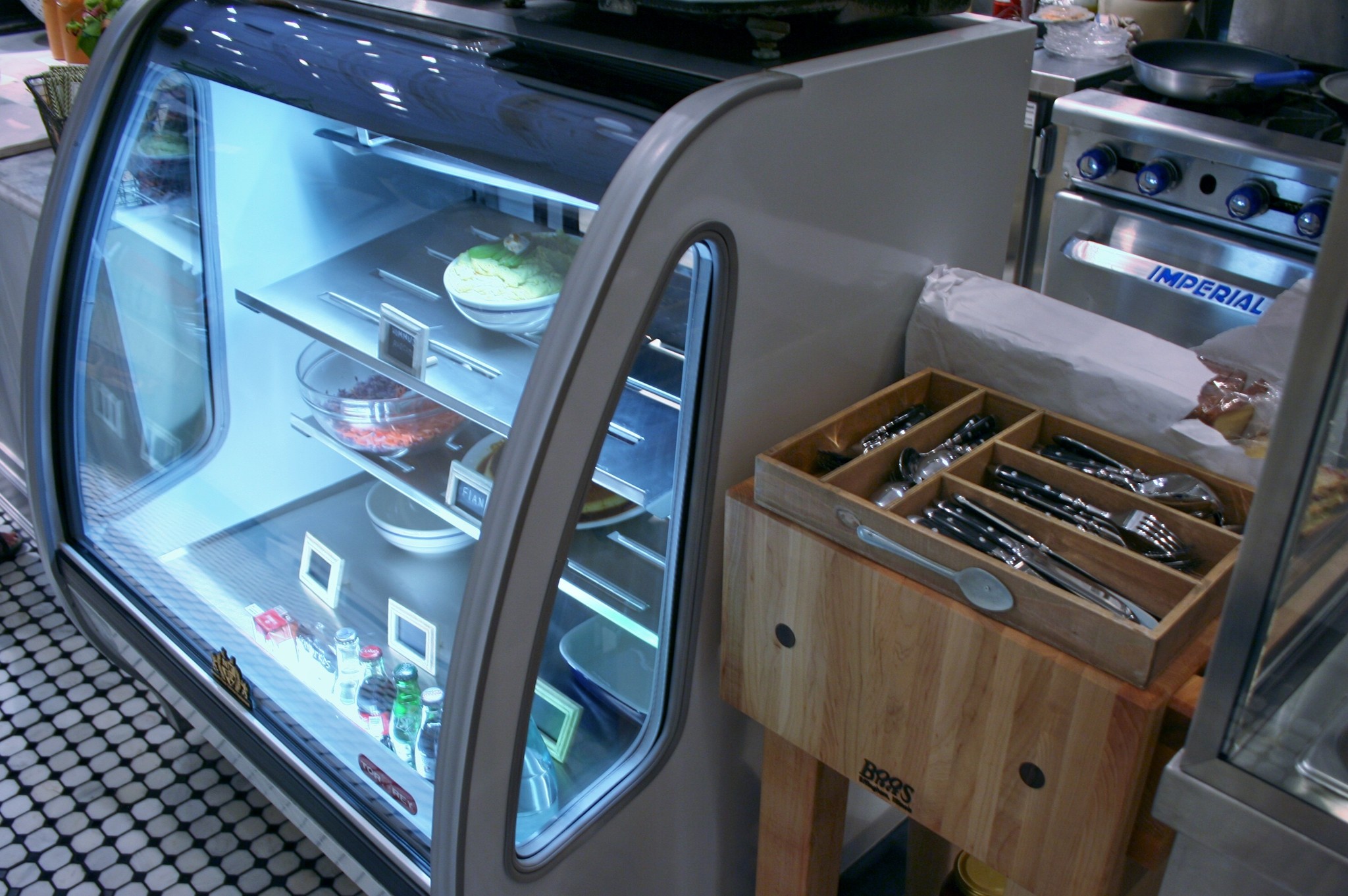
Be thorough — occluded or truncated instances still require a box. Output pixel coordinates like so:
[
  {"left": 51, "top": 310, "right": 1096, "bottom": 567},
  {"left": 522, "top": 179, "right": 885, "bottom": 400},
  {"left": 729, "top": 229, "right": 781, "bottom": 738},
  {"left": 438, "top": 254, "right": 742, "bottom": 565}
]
[
  {"left": 440, "top": 233, "right": 582, "bottom": 333},
  {"left": 366, "top": 480, "right": 475, "bottom": 557},
  {"left": 291, "top": 340, "right": 473, "bottom": 454},
  {"left": 128, "top": 129, "right": 190, "bottom": 186}
]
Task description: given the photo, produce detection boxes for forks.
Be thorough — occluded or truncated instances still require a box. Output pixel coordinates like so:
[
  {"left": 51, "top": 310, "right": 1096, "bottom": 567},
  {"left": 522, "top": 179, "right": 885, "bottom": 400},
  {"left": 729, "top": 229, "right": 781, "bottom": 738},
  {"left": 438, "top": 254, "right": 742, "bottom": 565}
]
[{"left": 988, "top": 465, "right": 1193, "bottom": 577}]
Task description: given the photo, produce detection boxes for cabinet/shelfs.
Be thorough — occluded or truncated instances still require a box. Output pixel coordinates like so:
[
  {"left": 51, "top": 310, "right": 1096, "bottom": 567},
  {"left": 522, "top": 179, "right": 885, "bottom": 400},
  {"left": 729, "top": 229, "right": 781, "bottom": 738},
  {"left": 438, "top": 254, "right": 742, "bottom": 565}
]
[{"left": 18, "top": 1, "right": 1040, "bottom": 895}]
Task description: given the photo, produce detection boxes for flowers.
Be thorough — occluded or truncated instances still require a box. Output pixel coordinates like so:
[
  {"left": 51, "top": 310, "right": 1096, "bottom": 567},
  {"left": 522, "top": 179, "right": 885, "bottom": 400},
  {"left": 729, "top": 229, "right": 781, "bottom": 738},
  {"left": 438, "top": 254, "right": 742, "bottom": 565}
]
[{"left": 64, "top": 0, "right": 127, "bottom": 58}]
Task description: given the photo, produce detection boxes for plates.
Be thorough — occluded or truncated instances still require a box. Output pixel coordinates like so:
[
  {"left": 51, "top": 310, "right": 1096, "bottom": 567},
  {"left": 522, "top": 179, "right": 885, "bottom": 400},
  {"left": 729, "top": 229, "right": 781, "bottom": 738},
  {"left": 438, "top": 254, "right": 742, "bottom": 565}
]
[
  {"left": 559, "top": 614, "right": 656, "bottom": 724},
  {"left": 1321, "top": 69, "right": 1348, "bottom": 107},
  {"left": 462, "top": 430, "right": 648, "bottom": 531}
]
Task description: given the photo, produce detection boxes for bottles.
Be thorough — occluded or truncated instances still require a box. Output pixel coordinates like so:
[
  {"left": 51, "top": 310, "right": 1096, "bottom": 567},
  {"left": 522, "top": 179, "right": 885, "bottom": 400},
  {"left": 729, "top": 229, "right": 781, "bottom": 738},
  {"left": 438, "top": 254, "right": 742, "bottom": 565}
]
[{"left": 330, "top": 627, "right": 560, "bottom": 846}]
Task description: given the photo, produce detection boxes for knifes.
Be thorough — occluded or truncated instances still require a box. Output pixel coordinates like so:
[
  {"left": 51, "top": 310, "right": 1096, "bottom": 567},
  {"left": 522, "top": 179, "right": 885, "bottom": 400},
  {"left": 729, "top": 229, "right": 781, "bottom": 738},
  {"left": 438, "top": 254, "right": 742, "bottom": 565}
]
[{"left": 905, "top": 493, "right": 1162, "bottom": 631}]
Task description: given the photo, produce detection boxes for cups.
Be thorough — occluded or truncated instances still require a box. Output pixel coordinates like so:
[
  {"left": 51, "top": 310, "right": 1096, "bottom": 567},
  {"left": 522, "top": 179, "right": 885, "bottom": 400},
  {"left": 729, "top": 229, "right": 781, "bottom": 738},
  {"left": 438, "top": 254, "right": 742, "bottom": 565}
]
[{"left": 42, "top": 0, "right": 94, "bottom": 65}]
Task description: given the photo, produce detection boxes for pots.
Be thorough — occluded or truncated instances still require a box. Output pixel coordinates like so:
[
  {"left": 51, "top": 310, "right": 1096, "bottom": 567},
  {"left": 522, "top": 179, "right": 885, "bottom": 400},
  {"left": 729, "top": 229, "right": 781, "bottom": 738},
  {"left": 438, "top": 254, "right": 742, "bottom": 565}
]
[
  {"left": 1130, "top": 39, "right": 1314, "bottom": 105},
  {"left": 1097, "top": 0, "right": 1192, "bottom": 45}
]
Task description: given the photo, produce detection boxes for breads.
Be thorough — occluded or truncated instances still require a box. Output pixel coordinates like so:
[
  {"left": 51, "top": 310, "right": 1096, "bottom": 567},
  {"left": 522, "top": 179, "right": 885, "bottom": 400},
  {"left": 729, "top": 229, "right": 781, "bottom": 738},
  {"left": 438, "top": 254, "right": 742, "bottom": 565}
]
[{"left": 1188, "top": 356, "right": 1347, "bottom": 538}]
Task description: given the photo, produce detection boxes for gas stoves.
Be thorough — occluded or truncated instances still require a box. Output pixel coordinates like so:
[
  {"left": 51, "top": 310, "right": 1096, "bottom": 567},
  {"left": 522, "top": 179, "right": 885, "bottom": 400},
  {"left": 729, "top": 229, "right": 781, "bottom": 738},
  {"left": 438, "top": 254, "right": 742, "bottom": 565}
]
[{"left": 1035, "top": 80, "right": 1348, "bottom": 253}]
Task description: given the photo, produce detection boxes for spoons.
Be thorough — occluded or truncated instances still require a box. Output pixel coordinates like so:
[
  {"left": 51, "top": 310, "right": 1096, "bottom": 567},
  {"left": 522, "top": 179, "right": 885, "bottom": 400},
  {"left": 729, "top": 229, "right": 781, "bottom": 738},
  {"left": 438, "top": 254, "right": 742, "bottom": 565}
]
[
  {"left": 1035, "top": 433, "right": 1229, "bottom": 529},
  {"left": 857, "top": 526, "right": 1016, "bottom": 616},
  {"left": 868, "top": 416, "right": 1006, "bottom": 511}
]
